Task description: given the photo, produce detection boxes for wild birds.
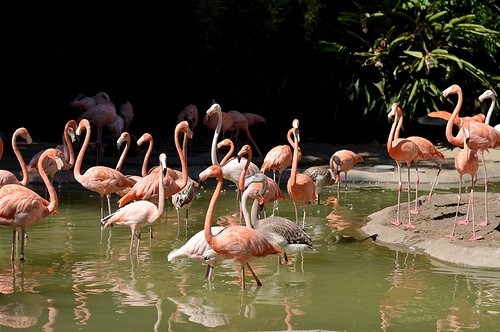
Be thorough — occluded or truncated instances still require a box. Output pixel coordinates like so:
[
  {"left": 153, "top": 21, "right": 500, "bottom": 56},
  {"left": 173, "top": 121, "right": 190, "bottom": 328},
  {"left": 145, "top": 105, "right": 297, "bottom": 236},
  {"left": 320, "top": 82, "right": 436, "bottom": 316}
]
[
  {"left": 387, "top": 103, "right": 445, "bottom": 229},
  {"left": 0, "top": 119, "right": 77, "bottom": 259},
  {"left": 428, "top": 84, "right": 497, "bottom": 242},
  {"left": 72, "top": 92, "right": 364, "bottom": 290}
]
